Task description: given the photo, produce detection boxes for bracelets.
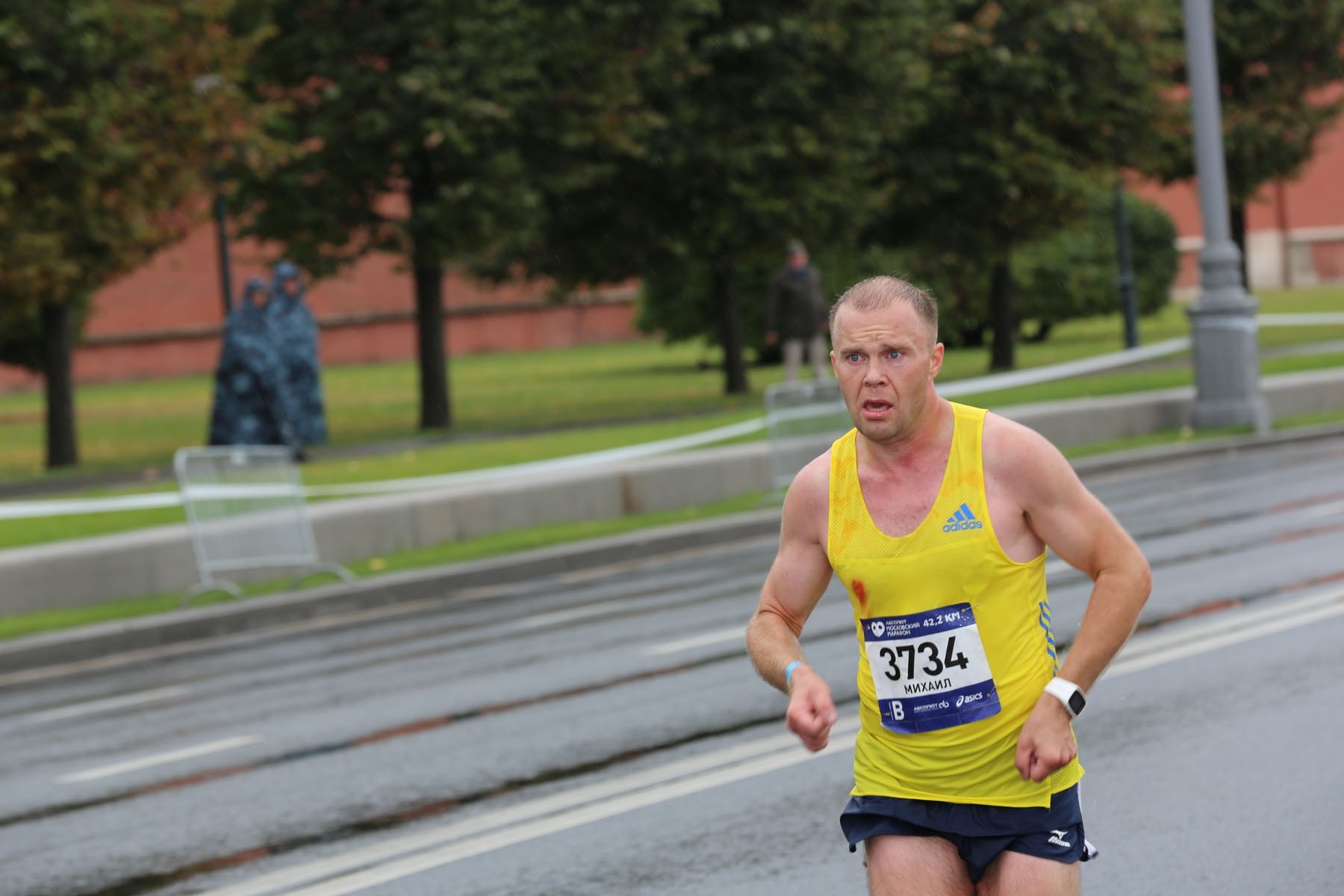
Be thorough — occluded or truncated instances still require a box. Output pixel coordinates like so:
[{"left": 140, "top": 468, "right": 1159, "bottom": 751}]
[{"left": 786, "top": 661, "right": 803, "bottom": 689}]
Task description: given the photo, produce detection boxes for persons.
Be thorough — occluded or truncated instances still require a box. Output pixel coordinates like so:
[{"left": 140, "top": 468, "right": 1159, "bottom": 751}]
[
  {"left": 204, "top": 276, "right": 305, "bottom": 460},
  {"left": 760, "top": 240, "right": 839, "bottom": 401},
  {"left": 266, "top": 259, "right": 326, "bottom": 449},
  {"left": 747, "top": 274, "right": 1153, "bottom": 895}
]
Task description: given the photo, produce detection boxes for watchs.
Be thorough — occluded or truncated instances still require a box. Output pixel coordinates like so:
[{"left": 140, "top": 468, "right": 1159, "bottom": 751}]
[{"left": 1044, "top": 674, "right": 1089, "bottom": 720}]
